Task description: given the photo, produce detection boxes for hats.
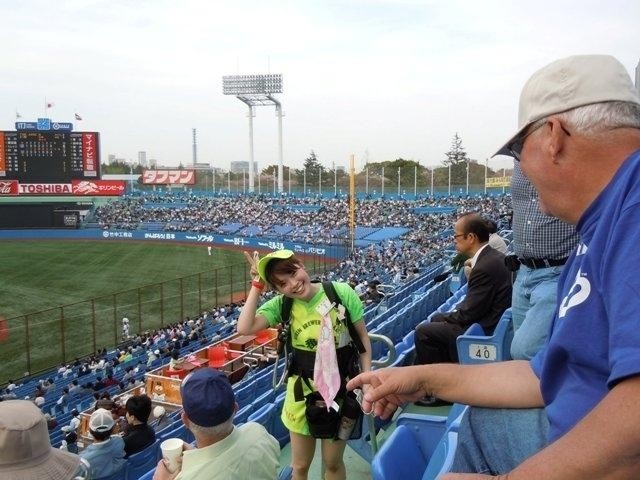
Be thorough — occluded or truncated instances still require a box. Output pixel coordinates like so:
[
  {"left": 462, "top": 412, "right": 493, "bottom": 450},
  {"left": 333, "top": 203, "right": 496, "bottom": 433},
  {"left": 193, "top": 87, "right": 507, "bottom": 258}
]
[
  {"left": 0, "top": 400, "right": 81, "bottom": 480},
  {"left": 89, "top": 408, "right": 115, "bottom": 433},
  {"left": 257, "top": 249, "right": 295, "bottom": 286},
  {"left": 180, "top": 368, "right": 234, "bottom": 427},
  {"left": 489, "top": 55, "right": 640, "bottom": 159}
]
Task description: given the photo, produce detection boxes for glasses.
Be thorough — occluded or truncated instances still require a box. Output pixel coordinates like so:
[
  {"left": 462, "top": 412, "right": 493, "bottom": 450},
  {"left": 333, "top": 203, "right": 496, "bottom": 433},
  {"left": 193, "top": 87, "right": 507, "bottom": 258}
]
[
  {"left": 506, "top": 121, "right": 572, "bottom": 162},
  {"left": 453, "top": 232, "right": 470, "bottom": 239}
]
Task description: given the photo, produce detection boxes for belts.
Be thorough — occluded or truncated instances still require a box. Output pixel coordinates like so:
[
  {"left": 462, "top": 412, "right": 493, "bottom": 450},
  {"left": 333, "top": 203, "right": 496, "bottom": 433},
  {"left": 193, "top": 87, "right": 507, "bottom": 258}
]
[{"left": 516, "top": 257, "right": 569, "bottom": 270}]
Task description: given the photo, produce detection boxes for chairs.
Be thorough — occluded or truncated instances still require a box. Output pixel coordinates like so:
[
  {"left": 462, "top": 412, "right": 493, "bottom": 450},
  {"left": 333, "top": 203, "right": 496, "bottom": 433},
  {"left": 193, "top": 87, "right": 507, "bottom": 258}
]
[
  {"left": 1, "top": 240, "right": 517, "bottom": 480},
  {"left": 94, "top": 180, "right": 516, "bottom": 238}
]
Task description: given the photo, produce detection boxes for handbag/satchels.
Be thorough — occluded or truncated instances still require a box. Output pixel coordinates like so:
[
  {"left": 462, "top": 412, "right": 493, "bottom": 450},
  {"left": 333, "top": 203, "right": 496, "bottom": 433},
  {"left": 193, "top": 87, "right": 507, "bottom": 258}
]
[{"left": 307, "top": 392, "right": 340, "bottom": 439}]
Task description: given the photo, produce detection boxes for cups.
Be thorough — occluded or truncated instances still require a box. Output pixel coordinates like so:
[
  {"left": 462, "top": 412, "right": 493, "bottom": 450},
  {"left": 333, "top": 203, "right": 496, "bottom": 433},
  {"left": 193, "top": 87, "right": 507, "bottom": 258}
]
[{"left": 160, "top": 438, "right": 183, "bottom": 474}]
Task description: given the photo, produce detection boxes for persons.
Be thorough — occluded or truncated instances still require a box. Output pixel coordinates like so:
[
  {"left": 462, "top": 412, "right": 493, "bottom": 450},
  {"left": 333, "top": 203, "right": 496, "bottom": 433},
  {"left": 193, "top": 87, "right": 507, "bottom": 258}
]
[{"left": 1, "top": 52, "right": 639, "bottom": 479}]
